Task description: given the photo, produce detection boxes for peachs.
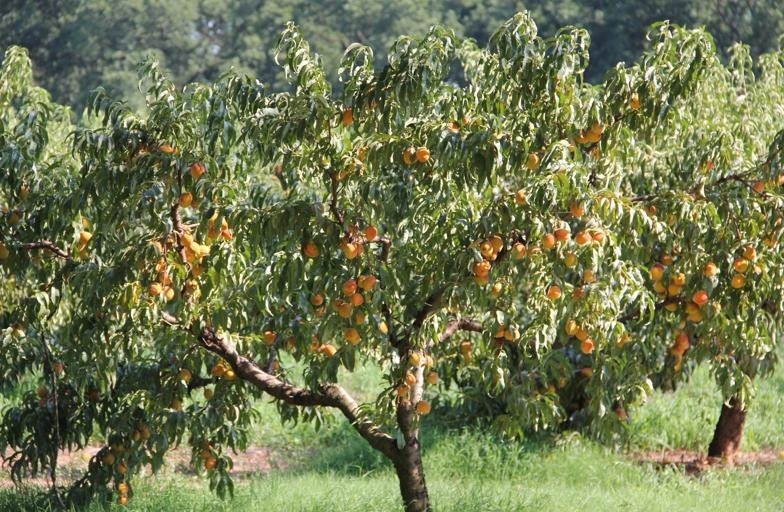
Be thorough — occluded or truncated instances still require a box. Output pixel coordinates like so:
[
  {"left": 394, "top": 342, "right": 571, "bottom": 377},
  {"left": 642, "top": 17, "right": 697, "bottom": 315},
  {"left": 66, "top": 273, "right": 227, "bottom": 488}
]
[{"left": 0, "top": 84, "right": 783, "bottom": 506}]
